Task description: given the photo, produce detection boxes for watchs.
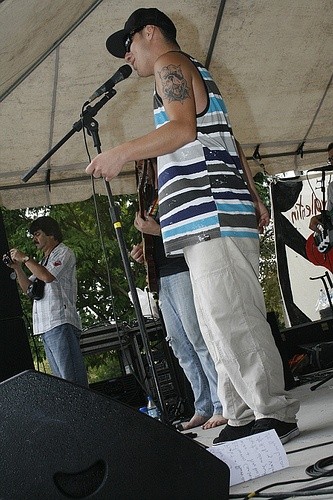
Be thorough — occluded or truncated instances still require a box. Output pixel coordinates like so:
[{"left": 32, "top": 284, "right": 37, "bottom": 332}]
[{"left": 22, "top": 256, "right": 29, "bottom": 262}]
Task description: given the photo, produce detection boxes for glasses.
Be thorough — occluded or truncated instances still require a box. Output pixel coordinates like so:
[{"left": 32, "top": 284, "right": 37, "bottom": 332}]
[{"left": 125, "top": 23, "right": 147, "bottom": 53}]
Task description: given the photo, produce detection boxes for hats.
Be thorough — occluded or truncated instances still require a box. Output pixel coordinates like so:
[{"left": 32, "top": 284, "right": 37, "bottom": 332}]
[{"left": 105, "top": 8, "right": 176, "bottom": 58}]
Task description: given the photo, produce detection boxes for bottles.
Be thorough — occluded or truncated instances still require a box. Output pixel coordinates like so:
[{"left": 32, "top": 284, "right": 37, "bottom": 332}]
[
  {"left": 139, "top": 407, "right": 149, "bottom": 416},
  {"left": 147, "top": 396, "right": 161, "bottom": 422},
  {"left": 320, "top": 287, "right": 333, "bottom": 307}
]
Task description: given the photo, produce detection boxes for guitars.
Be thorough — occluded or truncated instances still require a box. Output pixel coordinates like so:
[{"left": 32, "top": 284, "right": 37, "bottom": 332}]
[
  {"left": 136, "top": 159, "right": 159, "bottom": 293},
  {"left": 313, "top": 208, "right": 333, "bottom": 254}
]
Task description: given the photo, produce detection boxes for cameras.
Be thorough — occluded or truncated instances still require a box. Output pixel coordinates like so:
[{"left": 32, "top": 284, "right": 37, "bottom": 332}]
[{"left": 4, "top": 253, "right": 12, "bottom": 265}]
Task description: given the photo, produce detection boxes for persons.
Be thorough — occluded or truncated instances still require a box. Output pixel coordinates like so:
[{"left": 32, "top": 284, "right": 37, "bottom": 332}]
[
  {"left": 309, "top": 143, "right": 333, "bottom": 245},
  {"left": 3, "top": 217, "right": 89, "bottom": 388},
  {"left": 129, "top": 157, "right": 229, "bottom": 430},
  {"left": 86, "top": 7, "right": 301, "bottom": 444}
]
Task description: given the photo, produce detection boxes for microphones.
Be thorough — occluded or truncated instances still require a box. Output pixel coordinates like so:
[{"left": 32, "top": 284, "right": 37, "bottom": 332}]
[
  {"left": 84, "top": 64, "right": 132, "bottom": 107},
  {"left": 309, "top": 165, "right": 333, "bottom": 172}
]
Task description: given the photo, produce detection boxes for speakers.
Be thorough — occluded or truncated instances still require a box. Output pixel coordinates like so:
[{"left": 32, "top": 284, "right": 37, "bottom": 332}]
[{"left": 0, "top": 368, "right": 231, "bottom": 500}]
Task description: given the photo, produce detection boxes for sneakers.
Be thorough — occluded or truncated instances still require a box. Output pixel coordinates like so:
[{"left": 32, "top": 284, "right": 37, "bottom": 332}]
[
  {"left": 250, "top": 415, "right": 299, "bottom": 445},
  {"left": 212, "top": 420, "right": 258, "bottom": 445}
]
